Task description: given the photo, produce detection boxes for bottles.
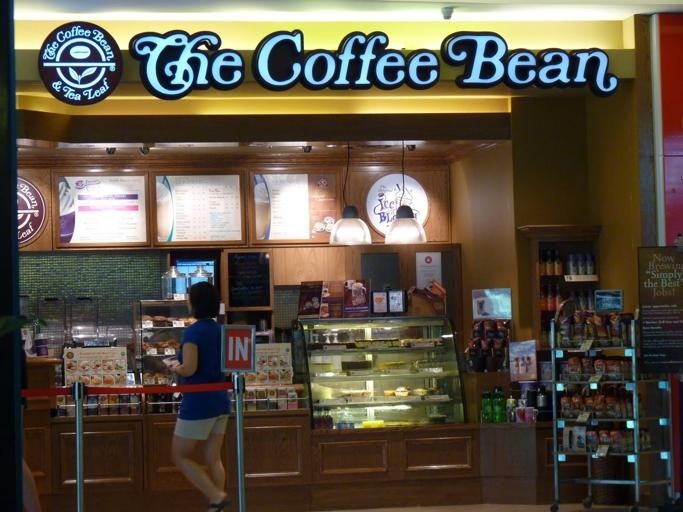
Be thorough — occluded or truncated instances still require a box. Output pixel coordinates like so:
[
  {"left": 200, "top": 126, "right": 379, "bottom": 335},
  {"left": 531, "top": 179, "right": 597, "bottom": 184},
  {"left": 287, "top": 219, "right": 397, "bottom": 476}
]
[
  {"left": 540, "top": 317, "right": 549, "bottom": 349},
  {"left": 539, "top": 282, "right": 595, "bottom": 310},
  {"left": 480, "top": 390, "right": 517, "bottom": 423},
  {"left": 538, "top": 249, "right": 563, "bottom": 277}
]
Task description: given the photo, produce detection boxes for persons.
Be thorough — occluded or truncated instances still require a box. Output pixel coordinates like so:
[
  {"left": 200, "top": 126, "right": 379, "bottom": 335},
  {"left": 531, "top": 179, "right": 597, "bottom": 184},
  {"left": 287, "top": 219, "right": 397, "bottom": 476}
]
[
  {"left": 19, "top": 342, "right": 46, "bottom": 511},
  {"left": 167, "top": 281, "right": 231, "bottom": 512}
]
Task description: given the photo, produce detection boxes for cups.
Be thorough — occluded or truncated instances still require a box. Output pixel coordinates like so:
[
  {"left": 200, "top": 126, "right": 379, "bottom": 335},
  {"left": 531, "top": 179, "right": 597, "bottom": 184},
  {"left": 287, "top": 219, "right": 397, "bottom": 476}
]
[{"left": 514, "top": 405, "right": 539, "bottom": 423}]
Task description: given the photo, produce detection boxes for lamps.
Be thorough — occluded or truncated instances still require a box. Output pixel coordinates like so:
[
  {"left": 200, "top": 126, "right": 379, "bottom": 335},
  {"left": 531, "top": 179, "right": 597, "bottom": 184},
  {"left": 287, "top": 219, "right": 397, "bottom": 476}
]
[{"left": 329, "top": 139, "right": 427, "bottom": 245}]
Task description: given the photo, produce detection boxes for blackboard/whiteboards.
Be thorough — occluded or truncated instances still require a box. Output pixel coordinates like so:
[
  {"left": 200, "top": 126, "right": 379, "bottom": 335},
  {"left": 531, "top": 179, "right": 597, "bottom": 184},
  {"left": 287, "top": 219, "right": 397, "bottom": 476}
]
[
  {"left": 224, "top": 248, "right": 273, "bottom": 311},
  {"left": 637, "top": 246, "right": 683, "bottom": 374}
]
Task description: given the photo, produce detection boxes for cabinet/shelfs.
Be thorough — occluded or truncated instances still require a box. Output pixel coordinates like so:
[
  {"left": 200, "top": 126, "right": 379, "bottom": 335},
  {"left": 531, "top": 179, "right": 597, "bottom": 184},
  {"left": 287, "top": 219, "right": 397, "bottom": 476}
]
[
  {"left": 133, "top": 299, "right": 196, "bottom": 389},
  {"left": 551, "top": 300, "right": 675, "bottom": 511},
  {"left": 518, "top": 225, "right": 600, "bottom": 349},
  {"left": 293, "top": 315, "right": 469, "bottom": 430}
]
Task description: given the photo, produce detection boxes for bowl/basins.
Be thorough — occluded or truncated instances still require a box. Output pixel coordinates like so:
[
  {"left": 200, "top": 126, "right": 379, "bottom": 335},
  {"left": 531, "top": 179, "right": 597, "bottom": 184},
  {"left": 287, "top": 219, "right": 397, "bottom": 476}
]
[{"left": 383, "top": 389, "right": 427, "bottom": 397}]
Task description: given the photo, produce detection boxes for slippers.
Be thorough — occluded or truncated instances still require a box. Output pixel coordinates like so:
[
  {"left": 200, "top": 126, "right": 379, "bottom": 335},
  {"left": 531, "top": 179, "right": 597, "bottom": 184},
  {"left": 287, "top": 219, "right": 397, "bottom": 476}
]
[{"left": 207, "top": 497, "right": 232, "bottom": 512}]
